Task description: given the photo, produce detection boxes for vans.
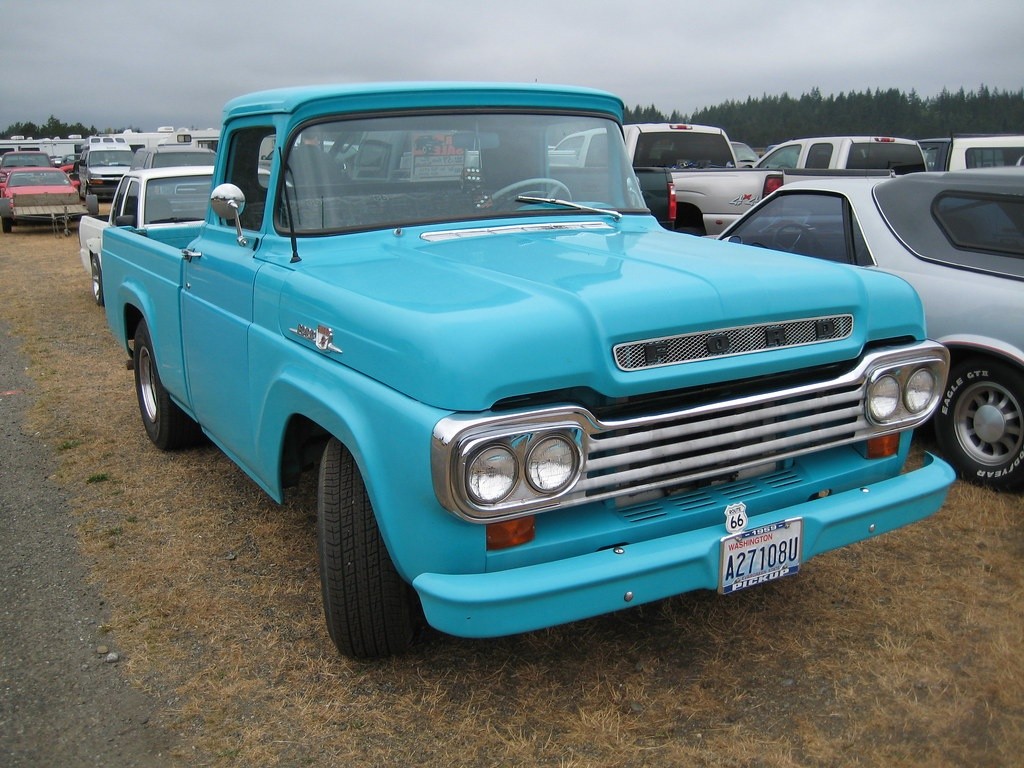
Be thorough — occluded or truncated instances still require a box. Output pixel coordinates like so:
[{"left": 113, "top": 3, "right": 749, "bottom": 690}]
[
  {"left": 130, "top": 144, "right": 217, "bottom": 212},
  {"left": 78, "top": 136, "right": 135, "bottom": 200}
]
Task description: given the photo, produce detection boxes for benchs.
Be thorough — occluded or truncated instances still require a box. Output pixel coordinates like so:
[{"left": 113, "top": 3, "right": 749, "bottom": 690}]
[{"left": 240, "top": 189, "right": 494, "bottom": 230}]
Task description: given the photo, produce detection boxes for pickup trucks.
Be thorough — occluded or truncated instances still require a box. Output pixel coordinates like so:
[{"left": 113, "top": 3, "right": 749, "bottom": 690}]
[
  {"left": 262, "top": 123, "right": 979, "bottom": 258},
  {"left": 79, "top": 165, "right": 294, "bottom": 306},
  {"left": 101, "top": 80, "right": 956, "bottom": 658}
]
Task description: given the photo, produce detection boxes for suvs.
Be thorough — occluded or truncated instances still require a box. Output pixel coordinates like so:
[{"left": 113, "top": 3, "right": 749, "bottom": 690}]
[{"left": 0, "top": 150, "right": 82, "bottom": 178}]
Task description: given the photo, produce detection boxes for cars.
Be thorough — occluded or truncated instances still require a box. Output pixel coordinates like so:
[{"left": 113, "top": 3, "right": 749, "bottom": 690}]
[
  {"left": 0, "top": 166, "right": 81, "bottom": 209},
  {"left": 717, "top": 133, "right": 1024, "bottom": 490}
]
